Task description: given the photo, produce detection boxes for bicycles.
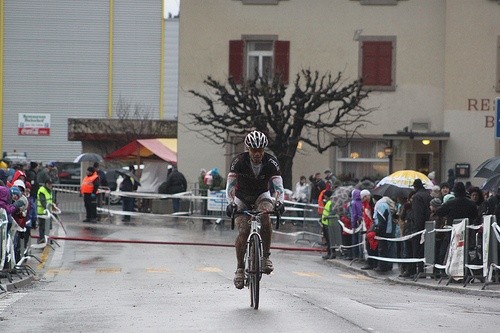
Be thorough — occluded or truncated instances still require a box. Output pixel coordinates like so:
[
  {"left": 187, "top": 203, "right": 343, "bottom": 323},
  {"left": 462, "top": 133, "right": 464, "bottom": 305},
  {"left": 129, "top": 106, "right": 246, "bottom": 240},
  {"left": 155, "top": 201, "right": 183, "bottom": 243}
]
[{"left": 230, "top": 201, "right": 283, "bottom": 310}]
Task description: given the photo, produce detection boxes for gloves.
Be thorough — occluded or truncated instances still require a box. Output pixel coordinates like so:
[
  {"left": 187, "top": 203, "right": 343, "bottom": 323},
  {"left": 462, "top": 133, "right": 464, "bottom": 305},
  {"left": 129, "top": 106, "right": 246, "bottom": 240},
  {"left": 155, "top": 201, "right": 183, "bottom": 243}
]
[
  {"left": 14, "top": 200, "right": 25, "bottom": 207},
  {"left": 275, "top": 201, "right": 284, "bottom": 214},
  {"left": 227, "top": 203, "right": 238, "bottom": 216}
]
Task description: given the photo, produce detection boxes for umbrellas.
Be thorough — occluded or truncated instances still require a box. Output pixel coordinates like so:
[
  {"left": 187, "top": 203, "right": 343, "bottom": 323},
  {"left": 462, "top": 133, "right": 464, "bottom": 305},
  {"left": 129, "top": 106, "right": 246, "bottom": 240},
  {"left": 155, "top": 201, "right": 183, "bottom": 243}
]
[
  {"left": 74, "top": 153, "right": 103, "bottom": 164},
  {"left": 375, "top": 169, "right": 436, "bottom": 190},
  {"left": 472, "top": 156, "right": 500, "bottom": 178},
  {"left": 480, "top": 173, "right": 500, "bottom": 193}
]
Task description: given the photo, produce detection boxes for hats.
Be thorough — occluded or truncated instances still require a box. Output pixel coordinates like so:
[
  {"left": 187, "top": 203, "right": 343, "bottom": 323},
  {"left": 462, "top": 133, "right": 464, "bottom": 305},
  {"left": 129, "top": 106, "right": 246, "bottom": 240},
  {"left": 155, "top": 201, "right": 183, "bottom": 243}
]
[
  {"left": 10, "top": 187, "right": 20, "bottom": 198},
  {"left": 411, "top": 179, "right": 425, "bottom": 186},
  {"left": 87, "top": 166, "right": 94, "bottom": 172},
  {"left": 31, "top": 162, "right": 38, "bottom": 169},
  {"left": 360, "top": 189, "right": 370, "bottom": 199},
  {"left": 430, "top": 198, "right": 442, "bottom": 207},
  {"left": 45, "top": 178, "right": 54, "bottom": 184}
]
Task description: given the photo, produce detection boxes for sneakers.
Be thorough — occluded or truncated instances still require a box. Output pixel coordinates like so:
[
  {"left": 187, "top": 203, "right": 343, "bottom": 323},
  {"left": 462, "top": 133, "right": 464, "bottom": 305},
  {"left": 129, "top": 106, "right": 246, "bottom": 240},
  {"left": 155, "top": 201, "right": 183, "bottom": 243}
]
[
  {"left": 263, "top": 258, "right": 274, "bottom": 272},
  {"left": 234, "top": 268, "right": 245, "bottom": 289}
]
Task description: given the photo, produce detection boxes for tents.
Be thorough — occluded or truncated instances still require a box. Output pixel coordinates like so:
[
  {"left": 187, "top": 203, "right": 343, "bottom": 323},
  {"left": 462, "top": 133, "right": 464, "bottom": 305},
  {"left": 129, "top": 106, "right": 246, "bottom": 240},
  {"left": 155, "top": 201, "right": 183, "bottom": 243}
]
[{"left": 104, "top": 138, "right": 176, "bottom": 164}]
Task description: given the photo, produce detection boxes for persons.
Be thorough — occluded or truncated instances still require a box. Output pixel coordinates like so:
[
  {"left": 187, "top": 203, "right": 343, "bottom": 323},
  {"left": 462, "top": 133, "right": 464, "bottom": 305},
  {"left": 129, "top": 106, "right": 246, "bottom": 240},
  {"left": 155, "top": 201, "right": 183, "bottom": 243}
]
[
  {"left": 165, "top": 167, "right": 187, "bottom": 220},
  {"left": 80, "top": 162, "right": 139, "bottom": 224},
  {"left": 0, "top": 160, "right": 59, "bottom": 278},
  {"left": 292, "top": 168, "right": 500, "bottom": 283},
  {"left": 198, "top": 170, "right": 227, "bottom": 226},
  {"left": 226, "top": 131, "right": 284, "bottom": 289}
]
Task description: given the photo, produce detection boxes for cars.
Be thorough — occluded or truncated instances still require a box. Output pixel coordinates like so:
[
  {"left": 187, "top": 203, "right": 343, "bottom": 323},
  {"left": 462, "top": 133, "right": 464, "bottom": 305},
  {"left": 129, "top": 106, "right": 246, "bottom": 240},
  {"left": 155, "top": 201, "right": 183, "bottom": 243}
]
[{"left": 46, "top": 160, "right": 141, "bottom": 205}]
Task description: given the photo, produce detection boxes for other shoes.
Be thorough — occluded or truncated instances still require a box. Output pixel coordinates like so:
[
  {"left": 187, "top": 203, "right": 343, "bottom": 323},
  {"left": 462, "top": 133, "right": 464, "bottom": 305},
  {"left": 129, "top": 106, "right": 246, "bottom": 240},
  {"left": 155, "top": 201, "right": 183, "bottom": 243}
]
[
  {"left": 83, "top": 219, "right": 90, "bottom": 222},
  {"left": 361, "top": 265, "right": 373, "bottom": 269},
  {"left": 399, "top": 272, "right": 411, "bottom": 276},
  {"left": 90, "top": 219, "right": 96, "bottom": 223},
  {"left": 373, "top": 267, "right": 387, "bottom": 271},
  {"left": 322, "top": 254, "right": 334, "bottom": 259}
]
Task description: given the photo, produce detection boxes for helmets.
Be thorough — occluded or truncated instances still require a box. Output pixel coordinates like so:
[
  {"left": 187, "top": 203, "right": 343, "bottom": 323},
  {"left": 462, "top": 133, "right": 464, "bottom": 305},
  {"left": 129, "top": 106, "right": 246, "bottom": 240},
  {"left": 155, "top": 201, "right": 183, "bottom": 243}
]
[{"left": 244, "top": 131, "right": 268, "bottom": 148}]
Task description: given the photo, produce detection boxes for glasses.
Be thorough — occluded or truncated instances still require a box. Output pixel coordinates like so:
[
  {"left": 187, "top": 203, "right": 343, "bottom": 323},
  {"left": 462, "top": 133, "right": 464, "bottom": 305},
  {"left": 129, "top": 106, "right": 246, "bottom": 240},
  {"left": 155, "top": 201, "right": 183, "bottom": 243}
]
[{"left": 249, "top": 148, "right": 264, "bottom": 153}]
